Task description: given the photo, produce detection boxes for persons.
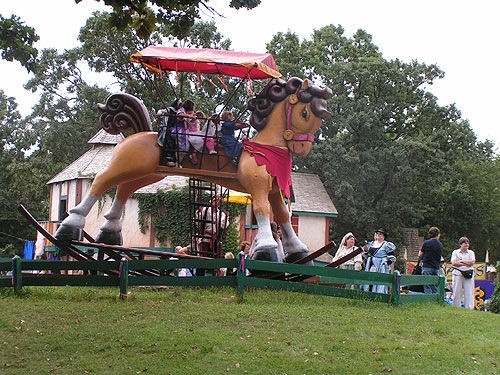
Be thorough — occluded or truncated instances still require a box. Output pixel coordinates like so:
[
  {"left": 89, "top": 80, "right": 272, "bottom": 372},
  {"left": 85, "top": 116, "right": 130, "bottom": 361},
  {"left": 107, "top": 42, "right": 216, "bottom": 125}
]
[
  {"left": 163, "top": 97, "right": 243, "bottom": 166},
  {"left": 196, "top": 196, "right": 229, "bottom": 274},
  {"left": 451, "top": 237, "right": 476, "bottom": 308},
  {"left": 31, "top": 224, "right": 136, "bottom": 277},
  {"left": 417, "top": 227, "right": 445, "bottom": 294},
  {"left": 169, "top": 224, "right": 286, "bottom": 276},
  {"left": 330, "top": 227, "right": 394, "bottom": 294}
]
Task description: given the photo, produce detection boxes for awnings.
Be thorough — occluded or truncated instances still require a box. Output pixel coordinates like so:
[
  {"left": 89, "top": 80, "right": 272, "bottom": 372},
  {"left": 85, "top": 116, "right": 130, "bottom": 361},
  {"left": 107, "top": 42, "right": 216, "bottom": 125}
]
[{"left": 130, "top": 45, "right": 281, "bottom": 119}]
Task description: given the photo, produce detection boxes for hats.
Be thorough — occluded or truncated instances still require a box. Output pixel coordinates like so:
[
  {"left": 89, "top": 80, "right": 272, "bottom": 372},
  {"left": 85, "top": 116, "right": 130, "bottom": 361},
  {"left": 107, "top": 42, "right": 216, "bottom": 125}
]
[{"left": 374, "top": 228, "right": 388, "bottom": 239}]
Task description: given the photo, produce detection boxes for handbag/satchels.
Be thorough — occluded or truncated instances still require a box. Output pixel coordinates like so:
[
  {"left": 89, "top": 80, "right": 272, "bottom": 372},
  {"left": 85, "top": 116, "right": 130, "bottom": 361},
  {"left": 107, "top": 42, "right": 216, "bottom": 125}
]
[
  {"left": 453, "top": 267, "right": 472, "bottom": 278},
  {"left": 408, "top": 265, "right": 423, "bottom": 292}
]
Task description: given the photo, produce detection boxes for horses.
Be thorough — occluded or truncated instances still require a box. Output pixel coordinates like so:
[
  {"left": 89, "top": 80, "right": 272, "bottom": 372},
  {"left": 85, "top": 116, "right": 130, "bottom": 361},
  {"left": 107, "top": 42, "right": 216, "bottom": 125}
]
[{"left": 55, "top": 75, "right": 334, "bottom": 267}]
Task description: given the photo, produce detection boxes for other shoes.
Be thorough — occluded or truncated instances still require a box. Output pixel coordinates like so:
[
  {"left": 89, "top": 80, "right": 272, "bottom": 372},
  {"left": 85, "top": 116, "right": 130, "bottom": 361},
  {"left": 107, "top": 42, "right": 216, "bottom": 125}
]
[
  {"left": 168, "top": 162, "right": 176, "bottom": 166},
  {"left": 210, "top": 150, "right": 217, "bottom": 154},
  {"left": 192, "top": 156, "right": 198, "bottom": 163},
  {"left": 231, "top": 158, "right": 237, "bottom": 164}
]
